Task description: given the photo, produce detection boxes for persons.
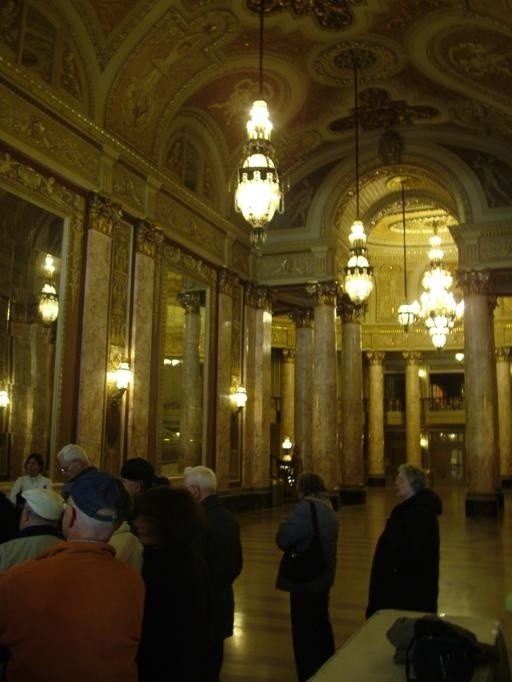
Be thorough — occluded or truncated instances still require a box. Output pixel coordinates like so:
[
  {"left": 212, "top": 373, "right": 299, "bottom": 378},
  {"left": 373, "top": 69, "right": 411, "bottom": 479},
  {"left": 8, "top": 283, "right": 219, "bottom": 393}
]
[
  {"left": 183, "top": 466, "right": 242, "bottom": 640},
  {"left": 0, "top": 486, "right": 68, "bottom": 576},
  {"left": 364, "top": 464, "right": 442, "bottom": 616},
  {"left": 56, "top": 445, "right": 91, "bottom": 479},
  {"left": 10, "top": 455, "right": 52, "bottom": 508},
  {"left": 0, "top": 470, "right": 147, "bottom": 681},
  {"left": 122, "top": 461, "right": 170, "bottom": 498},
  {"left": 135, "top": 487, "right": 224, "bottom": 682},
  {"left": 276, "top": 474, "right": 336, "bottom": 681}
]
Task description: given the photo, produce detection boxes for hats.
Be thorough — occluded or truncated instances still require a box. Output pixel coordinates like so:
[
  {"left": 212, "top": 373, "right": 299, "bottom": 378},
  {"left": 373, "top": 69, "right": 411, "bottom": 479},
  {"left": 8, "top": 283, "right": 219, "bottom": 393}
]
[
  {"left": 66, "top": 470, "right": 129, "bottom": 522},
  {"left": 120, "top": 457, "right": 154, "bottom": 483},
  {"left": 20, "top": 488, "right": 65, "bottom": 520}
]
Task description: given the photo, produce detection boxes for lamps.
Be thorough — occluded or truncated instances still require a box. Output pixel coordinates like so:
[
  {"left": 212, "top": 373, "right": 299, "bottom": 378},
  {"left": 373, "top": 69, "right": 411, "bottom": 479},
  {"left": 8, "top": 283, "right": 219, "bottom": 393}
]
[
  {"left": 411, "top": 220, "right": 465, "bottom": 348},
  {"left": 232, "top": 1, "right": 286, "bottom": 233},
  {"left": 343, "top": 58, "right": 375, "bottom": 305},
  {"left": 232, "top": 385, "right": 248, "bottom": 415},
  {"left": 37, "top": 253, "right": 60, "bottom": 325},
  {"left": 279, "top": 437, "right": 296, "bottom": 471},
  {"left": 397, "top": 180, "right": 415, "bottom": 335},
  {"left": 116, "top": 363, "right": 131, "bottom": 390}
]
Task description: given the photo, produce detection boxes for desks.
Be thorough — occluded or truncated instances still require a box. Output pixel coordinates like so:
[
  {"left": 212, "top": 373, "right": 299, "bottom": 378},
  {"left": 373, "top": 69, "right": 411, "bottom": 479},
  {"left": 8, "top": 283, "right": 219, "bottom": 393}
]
[{"left": 307, "top": 609, "right": 499, "bottom": 682}]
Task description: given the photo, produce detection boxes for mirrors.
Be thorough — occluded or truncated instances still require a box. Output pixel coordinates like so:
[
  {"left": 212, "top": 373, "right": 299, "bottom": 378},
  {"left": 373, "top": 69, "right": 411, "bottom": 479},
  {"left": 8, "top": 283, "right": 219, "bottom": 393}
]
[
  {"left": 156, "top": 269, "right": 213, "bottom": 478},
  {"left": 0, "top": 181, "right": 78, "bottom": 495}
]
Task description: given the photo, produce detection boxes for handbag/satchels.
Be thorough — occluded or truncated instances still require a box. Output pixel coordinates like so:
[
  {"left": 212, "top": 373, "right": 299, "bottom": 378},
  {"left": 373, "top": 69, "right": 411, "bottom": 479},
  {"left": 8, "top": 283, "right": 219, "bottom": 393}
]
[
  {"left": 406, "top": 616, "right": 476, "bottom": 682},
  {"left": 274, "top": 542, "right": 334, "bottom": 597}
]
